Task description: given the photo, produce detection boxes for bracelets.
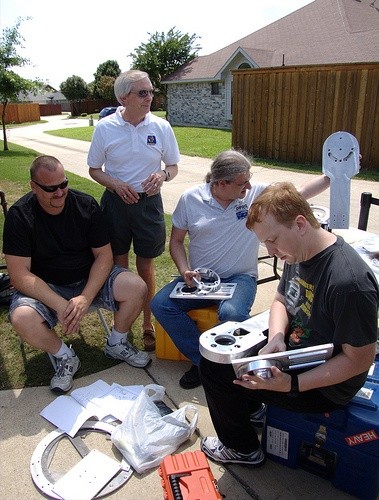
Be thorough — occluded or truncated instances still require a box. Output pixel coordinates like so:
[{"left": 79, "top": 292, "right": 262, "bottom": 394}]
[{"left": 290, "top": 373, "right": 300, "bottom": 393}]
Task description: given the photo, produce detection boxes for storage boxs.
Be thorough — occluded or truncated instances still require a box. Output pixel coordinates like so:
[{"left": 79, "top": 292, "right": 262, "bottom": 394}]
[
  {"left": 261, "top": 354, "right": 379, "bottom": 500},
  {"left": 156, "top": 305, "right": 219, "bottom": 363}
]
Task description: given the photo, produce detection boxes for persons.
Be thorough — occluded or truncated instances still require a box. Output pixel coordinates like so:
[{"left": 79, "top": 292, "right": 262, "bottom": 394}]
[
  {"left": 86, "top": 70, "right": 180, "bottom": 352},
  {"left": 201, "top": 182, "right": 379, "bottom": 465},
  {"left": 2, "top": 154, "right": 150, "bottom": 393},
  {"left": 150, "top": 147, "right": 363, "bottom": 391}
]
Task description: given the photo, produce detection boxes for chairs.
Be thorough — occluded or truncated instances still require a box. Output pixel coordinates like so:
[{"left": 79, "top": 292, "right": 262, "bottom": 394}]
[{"left": 0, "top": 191, "right": 111, "bottom": 373}]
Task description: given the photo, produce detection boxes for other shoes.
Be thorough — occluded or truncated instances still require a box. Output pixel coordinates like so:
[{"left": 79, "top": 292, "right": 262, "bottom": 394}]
[
  {"left": 142, "top": 324, "right": 156, "bottom": 348},
  {"left": 178, "top": 364, "right": 203, "bottom": 388}
]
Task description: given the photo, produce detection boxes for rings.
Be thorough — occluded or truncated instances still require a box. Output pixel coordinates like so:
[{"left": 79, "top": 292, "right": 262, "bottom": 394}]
[{"left": 124, "top": 194, "right": 128, "bottom": 198}]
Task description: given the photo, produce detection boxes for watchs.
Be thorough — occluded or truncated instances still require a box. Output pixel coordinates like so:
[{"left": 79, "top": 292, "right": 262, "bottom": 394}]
[{"left": 162, "top": 169, "right": 170, "bottom": 181}]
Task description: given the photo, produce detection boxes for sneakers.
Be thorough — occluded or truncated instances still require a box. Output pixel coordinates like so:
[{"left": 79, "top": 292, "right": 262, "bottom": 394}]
[
  {"left": 200, "top": 436, "right": 266, "bottom": 468},
  {"left": 48, "top": 344, "right": 81, "bottom": 392},
  {"left": 104, "top": 337, "right": 150, "bottom": 368},
  {"left": 249, "top": 402, "right": 267, "bottom": 427}
]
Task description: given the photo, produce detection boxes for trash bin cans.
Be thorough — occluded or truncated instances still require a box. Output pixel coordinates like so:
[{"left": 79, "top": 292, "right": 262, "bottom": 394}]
[{"left": 89, "top": 120, "right": 93, "bottom": 126}]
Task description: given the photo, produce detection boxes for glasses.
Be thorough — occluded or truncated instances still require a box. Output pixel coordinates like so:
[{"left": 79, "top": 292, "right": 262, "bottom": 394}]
[
  {"left": 30, "top": 175, "right": 68, "bottom": 193},
  {"left": 128, "top": 88, "right": 155, "bottom": 97}
]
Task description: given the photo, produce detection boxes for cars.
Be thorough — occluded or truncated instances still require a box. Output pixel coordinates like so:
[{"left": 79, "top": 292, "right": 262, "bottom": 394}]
[{"left": 97, "top": 107, "right": 117, "bottom": 120}]
